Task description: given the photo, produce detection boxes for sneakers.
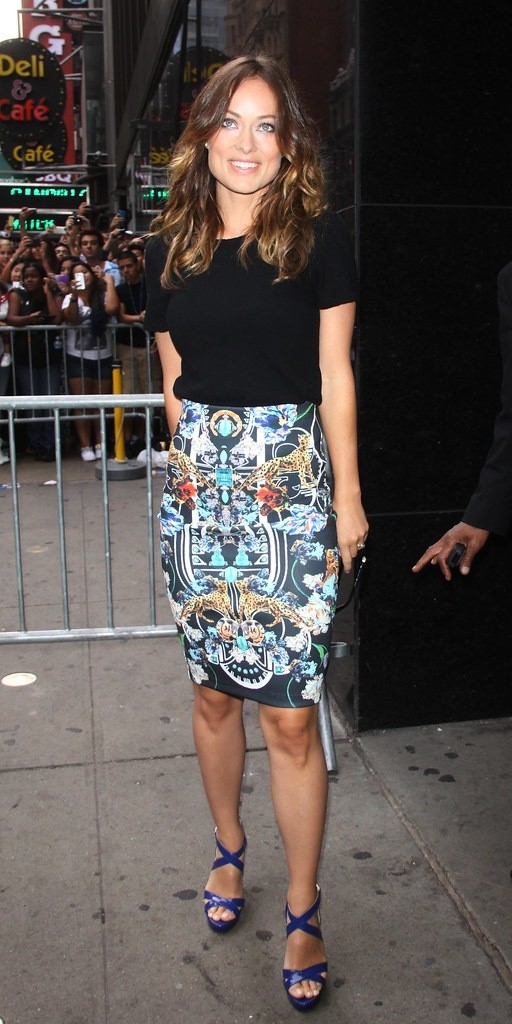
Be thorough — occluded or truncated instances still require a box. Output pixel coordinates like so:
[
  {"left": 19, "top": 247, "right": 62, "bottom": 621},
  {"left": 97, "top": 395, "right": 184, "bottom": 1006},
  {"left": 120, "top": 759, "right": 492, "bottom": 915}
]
[
  {"left": 0, "top": 352, "right": 12, "bottom": 367},
  {"left": 81, "top": 447, "right": 96, "bottom": 462},
  {"left": 95, "top": 444, "right": 103, "bottom": 459}
]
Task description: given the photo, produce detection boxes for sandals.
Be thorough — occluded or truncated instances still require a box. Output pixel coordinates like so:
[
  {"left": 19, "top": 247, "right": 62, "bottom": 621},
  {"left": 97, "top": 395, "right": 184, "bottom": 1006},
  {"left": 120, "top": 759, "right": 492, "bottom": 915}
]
[
  {"left": 203, "top": 825, "right": 247, "bottom": 934},
  {"left": 283, "top": 883, "right": 328, "bottom": 1011}
]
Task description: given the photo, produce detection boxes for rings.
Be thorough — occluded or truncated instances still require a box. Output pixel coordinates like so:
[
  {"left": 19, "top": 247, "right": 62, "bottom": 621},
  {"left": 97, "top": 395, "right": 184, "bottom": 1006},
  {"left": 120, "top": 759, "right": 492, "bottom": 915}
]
[{"left": 356, "top": 543, "right": 365, "bottom": 550}]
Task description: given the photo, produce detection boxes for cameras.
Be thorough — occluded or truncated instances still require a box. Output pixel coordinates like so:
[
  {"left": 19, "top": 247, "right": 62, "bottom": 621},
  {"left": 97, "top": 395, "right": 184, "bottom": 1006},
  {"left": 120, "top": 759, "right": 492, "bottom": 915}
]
[
  {"left": 73, "top": 217, "right": 83, "bottom": 225},
  {"left": 118, "top": 229, "right": 133, "bottom": 236}
]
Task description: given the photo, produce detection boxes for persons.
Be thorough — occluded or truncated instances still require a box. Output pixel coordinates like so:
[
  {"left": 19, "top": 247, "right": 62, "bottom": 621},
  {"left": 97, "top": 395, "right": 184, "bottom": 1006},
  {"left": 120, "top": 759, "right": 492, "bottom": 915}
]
[
  {"left": 412, "top": 407, "right": 512, "bottom": 587},
  {"left": 144, "top": 53, "right": 369, "bottom": 1012},
  {"left": 1, "top": 201, "right": 170, "bottom": 469}
]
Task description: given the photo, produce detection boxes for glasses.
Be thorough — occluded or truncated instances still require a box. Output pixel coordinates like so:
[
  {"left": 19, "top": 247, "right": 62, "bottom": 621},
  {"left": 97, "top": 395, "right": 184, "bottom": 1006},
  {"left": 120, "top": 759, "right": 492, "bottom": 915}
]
[{"left": 335, "top": 550, "right": 367, "bottom": 614}]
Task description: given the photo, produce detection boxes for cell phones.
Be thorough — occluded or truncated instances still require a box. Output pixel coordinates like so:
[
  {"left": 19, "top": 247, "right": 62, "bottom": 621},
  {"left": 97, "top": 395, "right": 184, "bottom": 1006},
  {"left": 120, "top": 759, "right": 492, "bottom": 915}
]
[
  {"left": 445, "top": 546, "right": 466, "bottom": 566},
  {"left": 75, "top": 273, "right": 85, "bottom": 290},
  {"left": 25, "top": 239, "right": 41, "bottom": 248},
  {"left": 28, "top": 209, "right": 37, "bottom": 219},
  {"left": 118, "top": 210, "right": 126, "bottom": 218}
]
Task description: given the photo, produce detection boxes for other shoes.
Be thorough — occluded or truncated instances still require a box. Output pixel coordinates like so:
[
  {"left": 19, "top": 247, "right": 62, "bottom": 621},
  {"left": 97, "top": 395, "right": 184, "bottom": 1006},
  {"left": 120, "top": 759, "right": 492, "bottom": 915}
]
[
  {"left": 35, "top": 441, "right": 67, "bottom": 461},
  {"left": 0, "top": 448, "right": 10, "bottom": 465}
]
